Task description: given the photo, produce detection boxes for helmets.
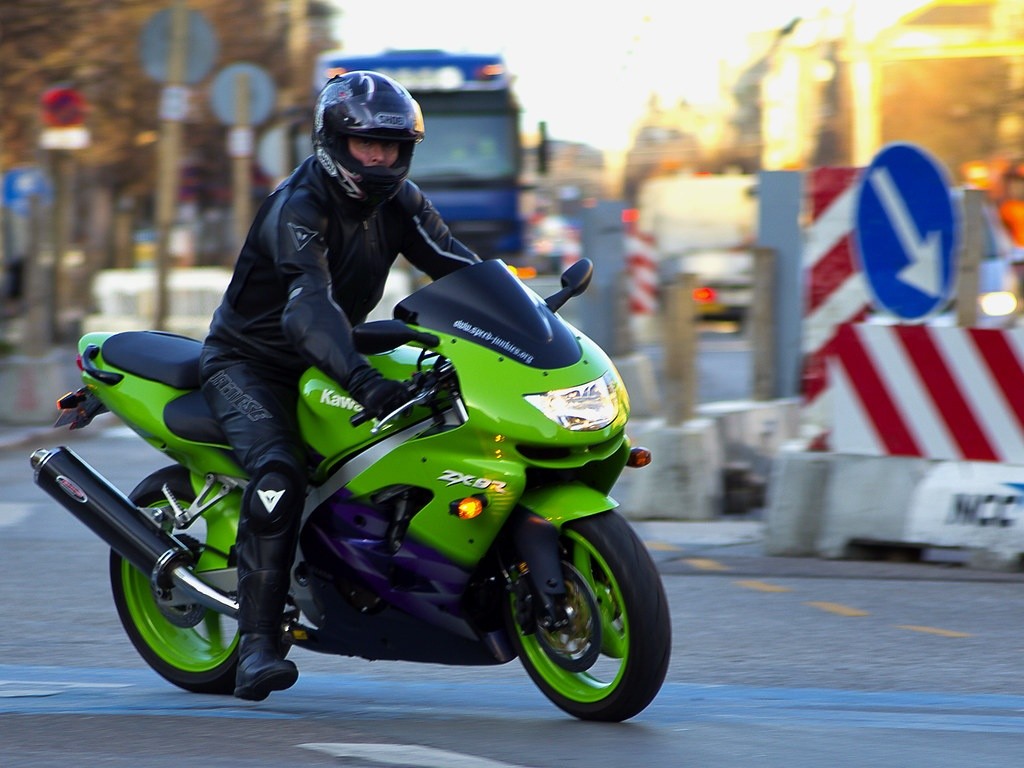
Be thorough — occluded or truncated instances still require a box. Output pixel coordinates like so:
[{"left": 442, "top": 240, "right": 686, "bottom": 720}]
[{"left": 312, "top": 70, "right": 422, "bottom": 212}]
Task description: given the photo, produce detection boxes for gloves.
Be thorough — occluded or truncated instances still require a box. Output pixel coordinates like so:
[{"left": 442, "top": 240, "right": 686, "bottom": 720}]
[{"left": 348, "top": 367, "right": 412, "bottom": 418}]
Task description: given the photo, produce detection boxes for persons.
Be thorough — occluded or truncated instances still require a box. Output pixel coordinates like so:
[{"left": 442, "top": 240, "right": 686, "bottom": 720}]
[{"left": 197, "top": 70, "right": 481, "bottom": 702}]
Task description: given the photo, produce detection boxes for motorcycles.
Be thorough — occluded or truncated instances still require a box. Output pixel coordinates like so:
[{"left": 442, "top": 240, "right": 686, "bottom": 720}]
[{"left": 30, "top": 258, "right": 673, "bottom": 724}]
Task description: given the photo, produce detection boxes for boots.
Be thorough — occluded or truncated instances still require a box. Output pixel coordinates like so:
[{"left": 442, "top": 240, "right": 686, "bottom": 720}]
[{"left": 234, "top": 449, "right": 306, "bottom": 701}]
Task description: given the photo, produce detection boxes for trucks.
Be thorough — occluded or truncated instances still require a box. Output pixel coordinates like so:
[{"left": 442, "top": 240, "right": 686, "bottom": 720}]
[{"left": 647, "top": 176, "right": 760, "bottom": 253}]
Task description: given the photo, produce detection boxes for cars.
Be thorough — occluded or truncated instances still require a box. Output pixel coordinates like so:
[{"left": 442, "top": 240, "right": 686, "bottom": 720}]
[
  {"left": 657, "top": 251, "right": 753, "bottom": 324},
  {"left": 523, "top": 215, "right": 581, "bottom": 275}
]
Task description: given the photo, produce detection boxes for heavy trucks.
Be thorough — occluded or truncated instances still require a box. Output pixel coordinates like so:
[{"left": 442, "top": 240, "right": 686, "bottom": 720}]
[{"left": 313, "top": 49, "right": 549, "bottom": 262}]
